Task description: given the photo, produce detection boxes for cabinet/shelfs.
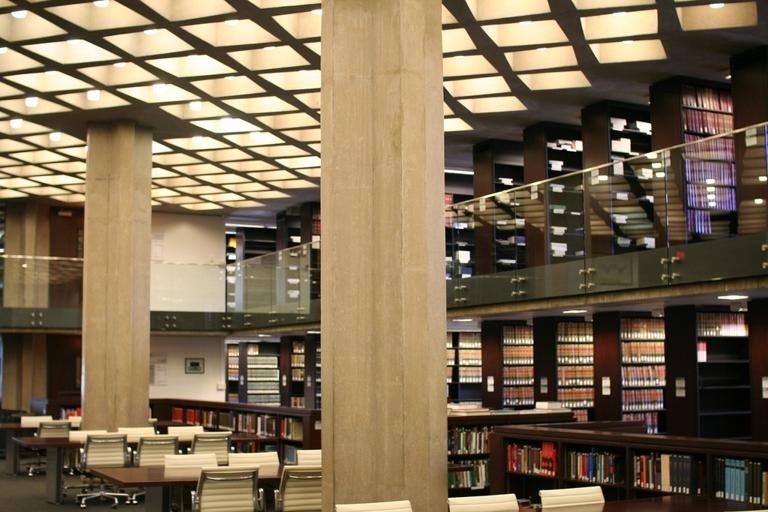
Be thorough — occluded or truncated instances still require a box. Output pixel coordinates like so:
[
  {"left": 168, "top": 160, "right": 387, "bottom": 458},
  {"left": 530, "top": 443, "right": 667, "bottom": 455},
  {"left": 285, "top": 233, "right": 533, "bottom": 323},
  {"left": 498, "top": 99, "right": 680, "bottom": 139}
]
[
  {"left": 173, "top": 309, "right": 767, "bottom": 511},
  {"left": 226, "top": 50, "right": 768, "bottom": 313}
]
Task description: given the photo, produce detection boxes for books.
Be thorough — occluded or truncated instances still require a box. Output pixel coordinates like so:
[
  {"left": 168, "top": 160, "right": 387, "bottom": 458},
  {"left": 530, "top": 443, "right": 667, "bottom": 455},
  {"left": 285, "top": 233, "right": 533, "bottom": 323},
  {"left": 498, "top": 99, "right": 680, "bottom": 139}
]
[
  {"left": 679, "top": 82, "right": 737, "bottom": 237},
  {"left": 61, "top": 405, "right": 82, "bottom": 419},
  {"left": 620, "top": 319, "right": 667, "bottom": 433},
  {"left": 697, "top": 342, "right": 706, "bottom": 362},
  {"left": 697, "top": 313, "right": 749, "bottom": 337},
  {"left": 557, "top": 322, "right": 594, "bottom": 421},
  {"left": 227, "top": 339, "right": 321, "bottom": 409},
  {"left": 503, "top": 440, "right": 767, "bottom": 510},
  {"left": 502, "top": 326, "right": 534, "bottom": 406},
  {"left": 448, "top": 401, "right": 571, "bottom": 415},
  {"left": 446, "top": 424, "right": 493, "bottom": 490},
  {"left": 446, "top": 332, "right": 483, "bottom": 382},
  {"left": 168, "top": 403, "right": 304, "bottom": 464}
]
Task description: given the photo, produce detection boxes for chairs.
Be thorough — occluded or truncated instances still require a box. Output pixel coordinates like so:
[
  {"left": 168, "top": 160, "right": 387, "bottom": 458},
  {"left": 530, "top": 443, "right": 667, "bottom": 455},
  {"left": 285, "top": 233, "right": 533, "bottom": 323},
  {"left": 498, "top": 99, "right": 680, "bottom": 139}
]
[
  {"left": 70, "top": 427, "right": 609, "bottom": 512},
  {"left": 20, "top": 416, "right": 54, "bottom": 475}
]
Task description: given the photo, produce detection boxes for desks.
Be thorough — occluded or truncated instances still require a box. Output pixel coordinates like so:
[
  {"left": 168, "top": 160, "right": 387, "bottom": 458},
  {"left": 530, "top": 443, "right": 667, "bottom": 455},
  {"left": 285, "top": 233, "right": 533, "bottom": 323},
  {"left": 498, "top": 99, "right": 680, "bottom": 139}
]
[
  {"left": 0, "top": 422, "right": 80, "bottom": 474},
  {"left": 11, "top": 432, "right": 281, "bottom": 503}
]
